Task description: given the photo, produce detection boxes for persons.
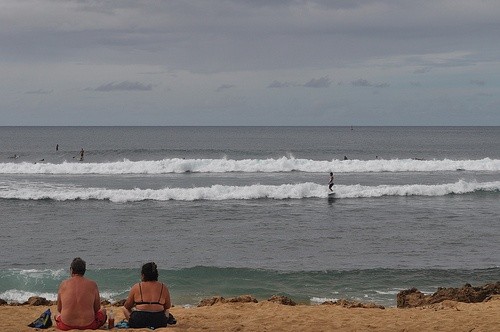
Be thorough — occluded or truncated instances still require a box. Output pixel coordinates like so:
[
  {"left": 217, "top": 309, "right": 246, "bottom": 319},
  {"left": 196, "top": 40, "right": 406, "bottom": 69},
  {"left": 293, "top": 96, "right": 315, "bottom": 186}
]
[
  {"left": 122, "top": 261, "right": 171, "bottom": 328},
  {"left": 328, "top": 172, "right": 334, "bottom": 192},
  {"left": 79, "top": 148, "right": 85, "bottom": 159},
  {"left": 54, "top": 257, "right": 107, "bottom": 331}
]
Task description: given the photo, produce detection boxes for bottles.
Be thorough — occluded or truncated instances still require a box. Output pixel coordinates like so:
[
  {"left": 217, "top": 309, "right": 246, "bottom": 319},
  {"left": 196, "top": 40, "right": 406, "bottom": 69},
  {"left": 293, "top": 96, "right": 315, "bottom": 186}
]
[{"left": 108, "top": 310, "right": 114, "bottom": 329}]
